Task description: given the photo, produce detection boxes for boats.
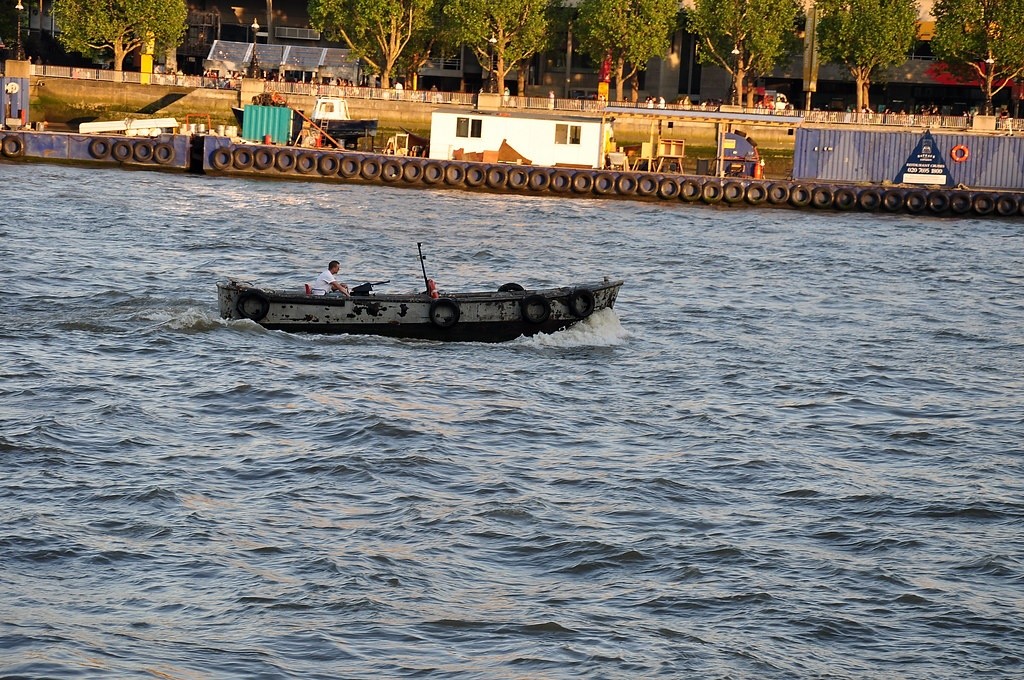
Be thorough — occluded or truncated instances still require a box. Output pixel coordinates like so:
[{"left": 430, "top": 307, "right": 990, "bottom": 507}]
[{"left": 215, "top": 276, "right": 626, "bottom": 343}]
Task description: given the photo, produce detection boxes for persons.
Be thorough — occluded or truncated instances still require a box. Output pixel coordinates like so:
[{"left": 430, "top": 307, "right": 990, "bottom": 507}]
[
  {"left": 658, "top": 97, "right": 665, "bottom": 110},
  {"left": 684, "top": 96, "right": 693, "bottom": 111},
  {"left": 312, "top": 260, "right": 350, "bottom": 296},
  {"left": 769, "top": 99, "right": 775, "bottom": 115},
  {"left": 154, "top": 64, "right": 161, "bottom": 82},
  {"left": 862, "top": 104, "right": 874, "bottom": 125},
  {"left": 757, "top": 99, "right": 763, "bottom": 113},
  {"left": 430, "top": 85, "right": 438, "bottom": 103},
  {"left": 1001, "top": 105, "right": 1010, "bottom": 130},
  {"left": 504, "top": 87, "right": 510, "bottom": 105},
  {"left": 647, "top": 96, "right": 657, "bottom": 109},
  {"left": 969, "top": 106, "right": 979, "bottom": 126},
  {"left": 920, "top": 105, "right": 939, "bottom": 116},
  {"left": 167, "top": 68, "right": 184, "bottom": 76},
  {"left": 549, "top": 90, "right": 554, "bottom": 109},
  {"left": 28, "top": 56, "right": 32, "bottom": 65},
  {"left": 396, "top": 82, "right": 403, "bottom": 98},
  {"left": 241, "top": 70, "right": 247, "bottom": 79}
]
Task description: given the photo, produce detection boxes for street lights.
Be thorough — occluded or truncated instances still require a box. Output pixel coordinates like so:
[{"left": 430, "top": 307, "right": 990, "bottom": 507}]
[
  {"left": 984, "top": 53, "right": 994, "bottom": 115},
  {"left": 730, "top": 44, "right": 739, "bottom": 106},
  {"left": 15, "top": 1, "right": 25, "bottom": 61},
  {"left": 246, "top": 18, "right": 261, "bottom": 79},
  {"left": 488, "top": 32, "right": 498, "bottom": 93}
]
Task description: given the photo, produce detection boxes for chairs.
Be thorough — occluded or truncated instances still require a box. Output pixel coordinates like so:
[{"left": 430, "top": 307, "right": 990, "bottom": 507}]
[{"left": 608, "top": 152, "right": 629, "bottom": 171}]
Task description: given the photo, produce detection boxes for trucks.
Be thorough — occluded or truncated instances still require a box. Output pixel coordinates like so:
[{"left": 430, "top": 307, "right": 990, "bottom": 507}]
[{"left": 739, "top": 87, "right": 789, "bottom": 111}]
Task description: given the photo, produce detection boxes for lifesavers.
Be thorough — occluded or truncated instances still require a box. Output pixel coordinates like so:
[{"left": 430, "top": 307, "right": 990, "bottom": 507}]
[
  {"left": 522, "top": 294, "right": 550, "bottom": 324},
  {"left": 255, "top": 149, "right": 275, "bottom": 170},
  {"left": 3, "top": 136, "right": 25, "bottom": 159},
  {"left": 235, "top": 288, "right": 270, "bottom": 323},
  {"left": 498, "top": 282, "right": 525, "bottom": 292},
  {"left": 884, "top": 191, "right": 1024, "bottom": 218},
  {"left": 430, "top": 297, "right": 460, "bottom": 328},
  {"left": 660, "top": 178, "right": 882, "bottom": 214},
  {"left": 212, "top": 145, "right": 233, "bottom": 171},
  {"left": 552, "top": 170, "right": 658, "bottom": 197},
  {"left": 382, "top": 159, "right": 550, "bottom": 194},
  {"left": 276, "top": 149, "right": 382, "bottom": 180},
  {"left": 234, "top": 148, "right": 254, "bottom": 169},
  {"left": 89, "top": 138, "right": 112, "bottom": 159},
  {"left": 569, "top": 289, "right": 595, "bottom": 318},
  {"left": 135, "top": 142, "right": 154, "bottom": 162},
  {"left": 113, "top": 140, "right": 133, "bottom": 161},
  {"left": 950, "top": 144, "right": 969, "bottom": 163},
  {"left": 154, "top": 143, "right": 175, "bottom": 164}
]
